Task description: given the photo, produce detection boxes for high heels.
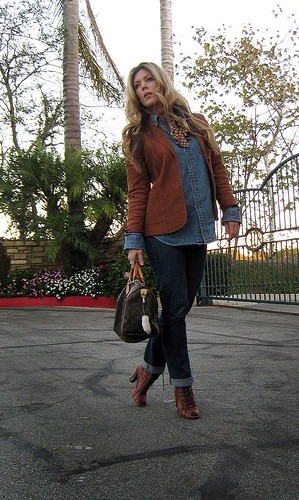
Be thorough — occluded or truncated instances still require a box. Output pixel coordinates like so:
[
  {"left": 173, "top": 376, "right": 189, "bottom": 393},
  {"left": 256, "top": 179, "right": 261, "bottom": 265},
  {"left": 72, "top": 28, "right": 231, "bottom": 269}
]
[
  {"left": 174, "top": 386, "right": 200, "bottom": 419},
  {"left": 129, "top": 361, "right": 161, "bottom": 407}
]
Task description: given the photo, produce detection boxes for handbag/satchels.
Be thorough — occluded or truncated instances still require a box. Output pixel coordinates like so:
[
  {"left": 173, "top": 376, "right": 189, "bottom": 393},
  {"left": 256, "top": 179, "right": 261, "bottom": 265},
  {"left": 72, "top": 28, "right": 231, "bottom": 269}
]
[{"left": 113, "top": 260, "right": 160, "bottom": 344}]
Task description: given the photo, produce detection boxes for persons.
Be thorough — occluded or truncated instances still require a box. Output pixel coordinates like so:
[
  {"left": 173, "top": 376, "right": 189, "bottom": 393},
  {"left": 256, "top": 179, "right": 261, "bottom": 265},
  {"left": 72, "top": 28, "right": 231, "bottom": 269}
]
[{"left": 120, "top": 61, "right": 241, "bottom": 419}]
